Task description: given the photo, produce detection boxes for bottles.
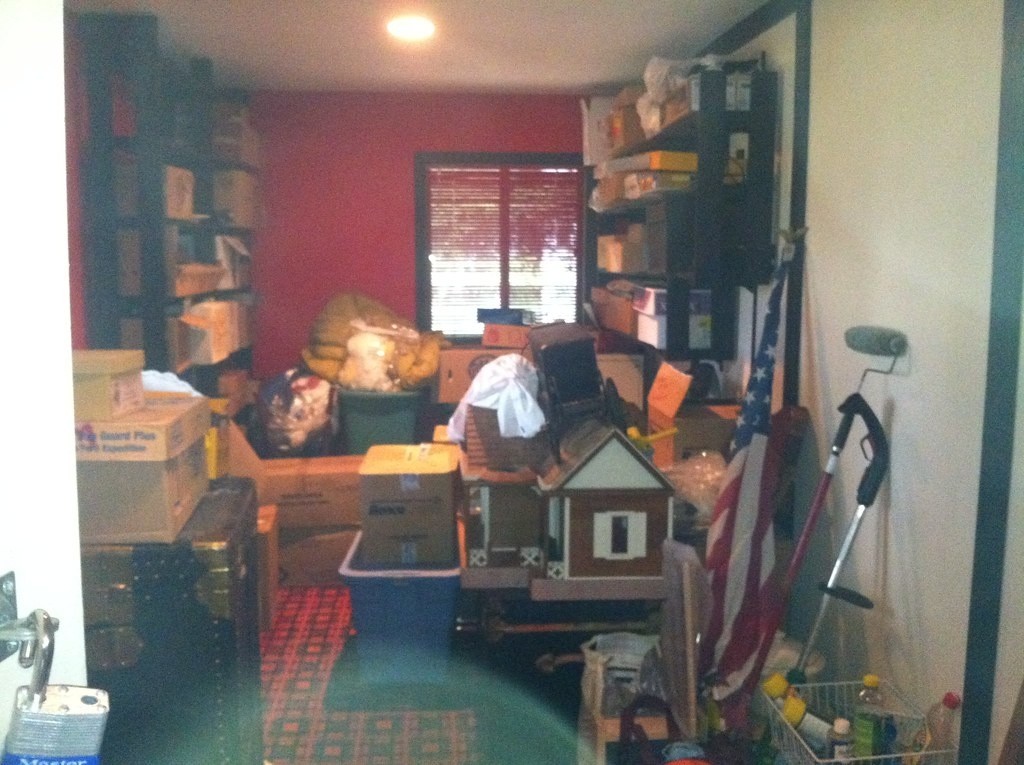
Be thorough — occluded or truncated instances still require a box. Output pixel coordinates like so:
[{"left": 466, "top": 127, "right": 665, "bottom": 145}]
[
  {"left": 827, "top": 718, "right": 854, "bottom": 759},
  {"left": 782, "top": 696, "right": 832, "bottom": 754},
  {"left": 904, "top": 692, "right": 961, "bottom": 765},
  {"left": 853, "top": 674, "right": 885, "bottom": 755},
  {"left": 764, "top": 673, "right": 799, "bottom": 709}
]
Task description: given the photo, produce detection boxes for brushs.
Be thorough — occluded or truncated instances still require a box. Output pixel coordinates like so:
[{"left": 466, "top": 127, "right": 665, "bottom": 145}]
[{"left": 726, "top": 325, "right": 910, "bottom": 731}]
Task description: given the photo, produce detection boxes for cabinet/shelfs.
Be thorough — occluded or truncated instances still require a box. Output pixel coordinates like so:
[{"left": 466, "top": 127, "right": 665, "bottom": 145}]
[
  {"left": 575, "top": 71, "right": 779, "bottom": 397},
  {"left": 86, "top": 474, "right": 263, "bottom": 764},
  {"left": 79, "top": 12, "right": 257, "bottom": 445}
]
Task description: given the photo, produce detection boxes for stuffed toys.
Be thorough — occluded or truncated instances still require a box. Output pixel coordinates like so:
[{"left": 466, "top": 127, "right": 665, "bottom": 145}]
[{"left": 337, "top": 313, "right": 419, "bottom": 392}]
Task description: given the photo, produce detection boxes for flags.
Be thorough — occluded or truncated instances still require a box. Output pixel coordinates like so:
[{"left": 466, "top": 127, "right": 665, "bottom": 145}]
[{"left": 698, "top": 226, "right": 810, "bottom": 737}]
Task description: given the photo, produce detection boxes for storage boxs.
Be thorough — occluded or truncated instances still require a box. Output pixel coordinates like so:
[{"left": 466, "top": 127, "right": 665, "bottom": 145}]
[
  {"left": 463, "top": 472, "right": 546, "bottom": 567},
  {"left": 336, "top": 528, "right": 463, "bottom": 688},
  {"left": 575, "top": 650, "right": 668, "bottom": 765},
  {"left": 261, "top": 455, "right": 363, "bottom": 530},
  {"left": 278, "top": 527, "right": 359, "bottom": 589},
  {"left": 113, "top": 71, "right": 784, "bottom": 469},
  {"left": 143, "top": 391, "right": 235, "bottom": 480},
  {"left": 74, "top": 347, "right": 211, "bottom": 544},
  {"left": 358, "top": 443, "right": 459, "bottom": 565},
  {"left": 257, "top": 504, "right": 282, "bottom": 631}
]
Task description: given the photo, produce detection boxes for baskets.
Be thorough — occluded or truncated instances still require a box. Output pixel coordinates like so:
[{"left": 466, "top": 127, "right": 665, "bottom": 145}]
[{"left": 758, "top": 677, "right": 959, "bottom": 765}]
